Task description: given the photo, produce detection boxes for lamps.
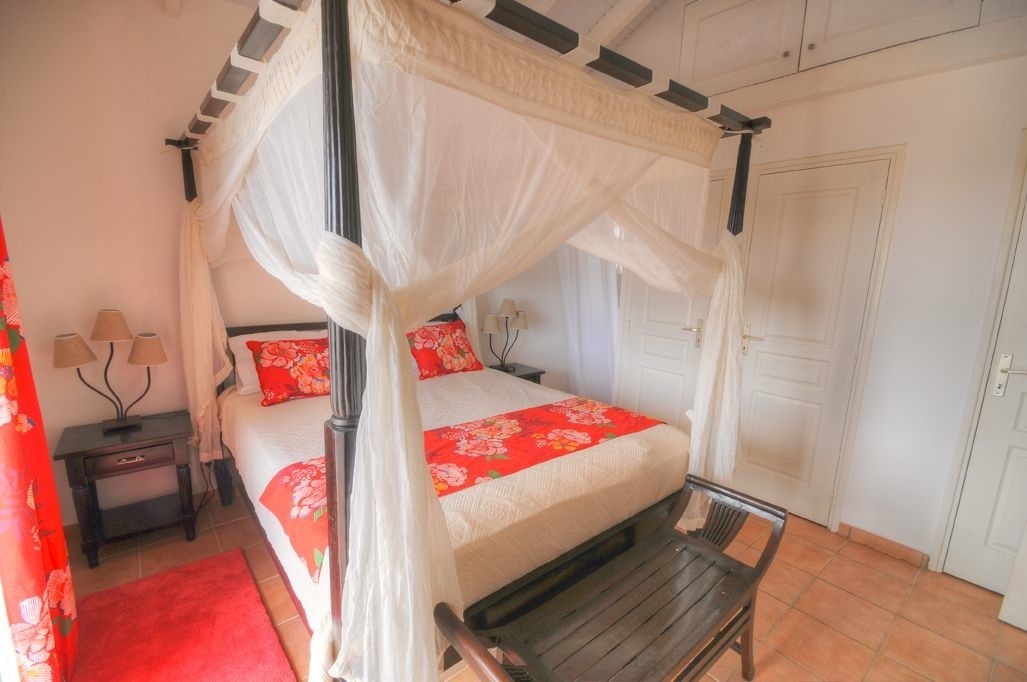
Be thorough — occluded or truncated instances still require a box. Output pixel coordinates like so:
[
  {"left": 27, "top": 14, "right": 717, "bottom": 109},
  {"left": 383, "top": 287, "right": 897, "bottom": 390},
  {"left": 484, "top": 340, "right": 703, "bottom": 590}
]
[
  {"left": 52, "top": 308, "right": 167, "bottom": 436},
  {"left": 482, "top": 299, "right": 529, "bottom": 372}
]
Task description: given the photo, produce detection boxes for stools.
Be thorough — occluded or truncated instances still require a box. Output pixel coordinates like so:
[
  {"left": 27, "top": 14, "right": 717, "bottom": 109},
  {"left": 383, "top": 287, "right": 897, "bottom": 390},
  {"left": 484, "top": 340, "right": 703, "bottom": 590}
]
[{"left": 433, "top": 475, "right": 789, "bottom": 682}]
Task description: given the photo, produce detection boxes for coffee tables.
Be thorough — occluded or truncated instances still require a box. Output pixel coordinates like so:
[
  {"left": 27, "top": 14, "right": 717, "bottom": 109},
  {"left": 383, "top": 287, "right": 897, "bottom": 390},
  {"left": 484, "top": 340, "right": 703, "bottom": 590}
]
[{"left": 207, "top": 315, "right": 689, "bottom": 682}]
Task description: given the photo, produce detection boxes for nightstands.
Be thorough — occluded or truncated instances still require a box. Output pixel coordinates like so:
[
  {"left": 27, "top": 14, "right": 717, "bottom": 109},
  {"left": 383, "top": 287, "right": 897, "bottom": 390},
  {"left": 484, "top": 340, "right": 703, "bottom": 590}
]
[
  {"left": 489, "top": 362, "right": 546, "bottom": 384},
  {"left": 53, "top": 409, "right": 195, "bottom": 569}
]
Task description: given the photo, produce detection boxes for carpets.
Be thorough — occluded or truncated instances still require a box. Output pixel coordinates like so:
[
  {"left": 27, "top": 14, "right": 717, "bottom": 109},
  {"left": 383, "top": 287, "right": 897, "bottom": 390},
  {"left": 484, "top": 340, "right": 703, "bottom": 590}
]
[{"left": 69, "top": 549, "right": 298, "bottom": 682}]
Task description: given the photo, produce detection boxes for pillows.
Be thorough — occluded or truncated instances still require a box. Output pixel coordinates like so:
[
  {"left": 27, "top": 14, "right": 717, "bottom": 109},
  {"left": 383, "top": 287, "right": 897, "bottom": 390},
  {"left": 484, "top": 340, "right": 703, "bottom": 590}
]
[
  {"left": 406, "top": 320, "right": 484, "bottom": 382},
  {"left": 246, "top": 336, "right": 332, "bottom": 407},
  {"left": 228, "top": 329, "right": 328, "bottom": 394}
]
